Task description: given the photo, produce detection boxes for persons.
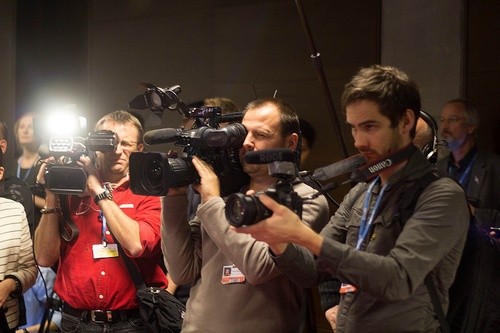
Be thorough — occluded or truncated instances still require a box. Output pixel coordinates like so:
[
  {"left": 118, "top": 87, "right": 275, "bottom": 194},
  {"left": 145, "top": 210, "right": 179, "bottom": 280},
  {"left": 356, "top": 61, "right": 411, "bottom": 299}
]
[
  {"left": 232, "top": 66, "right": 469, "bottom": 332},
  {"left": 412, "top": 98, "right": 499, "bottom": 261},
  {"left": 0, "top": 114, "right": 62, "bottom": 333},
  {"left": 34, "top": 109, "right": 162, "bottom": 332},
  {"left": 178, "top": 95, "right": 240, "bottom": 141},
  {"left": 163, "top": 97, "right": 328, "bottom": 332}
]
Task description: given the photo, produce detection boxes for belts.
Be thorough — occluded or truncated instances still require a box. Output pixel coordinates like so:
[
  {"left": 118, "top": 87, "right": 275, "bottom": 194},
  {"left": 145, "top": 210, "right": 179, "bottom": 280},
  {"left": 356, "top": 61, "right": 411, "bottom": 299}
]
[{"left": 62, "top": 301, "right": 143, "bottom": 323}]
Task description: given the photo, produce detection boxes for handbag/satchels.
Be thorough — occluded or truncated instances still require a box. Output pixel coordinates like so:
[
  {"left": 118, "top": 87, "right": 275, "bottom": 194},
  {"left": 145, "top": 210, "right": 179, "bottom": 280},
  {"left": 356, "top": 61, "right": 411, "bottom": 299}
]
[{"left": 135, "top": 286, "right": 186, "bottom": 333}]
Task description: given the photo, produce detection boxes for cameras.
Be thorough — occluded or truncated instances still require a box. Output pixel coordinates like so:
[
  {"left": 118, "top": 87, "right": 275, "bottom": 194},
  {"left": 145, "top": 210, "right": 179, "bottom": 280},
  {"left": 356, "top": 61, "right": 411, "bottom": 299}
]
[{"left": 225, "top": 148, "right": 309, "bottom": 228}]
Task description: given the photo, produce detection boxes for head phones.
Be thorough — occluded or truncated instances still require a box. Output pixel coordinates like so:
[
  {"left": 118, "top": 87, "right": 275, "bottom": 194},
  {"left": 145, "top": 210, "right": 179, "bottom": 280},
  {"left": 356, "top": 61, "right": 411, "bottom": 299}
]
[{"left": 419, "top": 110, "right": 437, "bottom": 164}]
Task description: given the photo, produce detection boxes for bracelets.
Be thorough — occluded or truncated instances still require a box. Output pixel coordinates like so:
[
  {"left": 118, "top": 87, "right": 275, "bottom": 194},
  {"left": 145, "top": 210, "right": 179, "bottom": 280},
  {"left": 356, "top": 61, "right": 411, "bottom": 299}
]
[{"left": 39, "top": 206, "right": 63, "bottom": 215}]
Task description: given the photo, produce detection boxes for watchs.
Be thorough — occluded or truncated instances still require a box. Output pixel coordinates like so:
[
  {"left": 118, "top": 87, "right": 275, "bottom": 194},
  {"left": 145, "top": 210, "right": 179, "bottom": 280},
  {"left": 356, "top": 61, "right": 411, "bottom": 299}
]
[{"left": 93, "top": 191, "right": 113, "bottom": 204}]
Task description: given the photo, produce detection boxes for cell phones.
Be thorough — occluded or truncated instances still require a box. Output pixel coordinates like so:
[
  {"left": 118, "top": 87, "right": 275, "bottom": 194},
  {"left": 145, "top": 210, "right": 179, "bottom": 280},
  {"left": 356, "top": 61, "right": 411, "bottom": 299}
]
[{"left": 489, "top": 227, "right": 500, "bottom": 238}]
